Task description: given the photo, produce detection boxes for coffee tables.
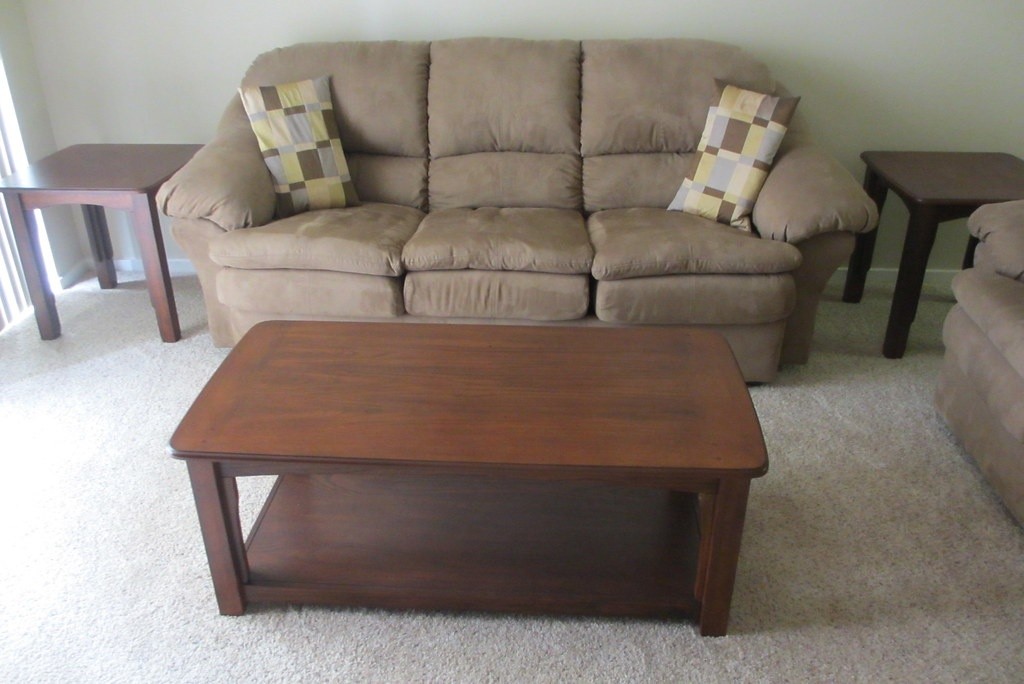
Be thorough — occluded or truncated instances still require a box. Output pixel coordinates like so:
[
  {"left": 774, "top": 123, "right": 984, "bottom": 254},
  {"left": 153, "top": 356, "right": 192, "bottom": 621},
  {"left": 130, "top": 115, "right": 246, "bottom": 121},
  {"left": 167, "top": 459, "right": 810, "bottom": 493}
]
[{"left": 161, "top": 319, "right": 771, "bottom": 639}]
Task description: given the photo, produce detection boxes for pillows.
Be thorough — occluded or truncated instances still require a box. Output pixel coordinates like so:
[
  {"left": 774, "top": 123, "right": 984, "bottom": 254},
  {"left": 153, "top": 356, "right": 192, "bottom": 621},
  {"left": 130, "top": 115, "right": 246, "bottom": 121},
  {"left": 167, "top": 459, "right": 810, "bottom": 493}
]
[
  {"left": 666, "top": 75, "right": 802, "bottom": 236},
  {"left": 235, "top": 73, "right": 365, "bottom": 220}
]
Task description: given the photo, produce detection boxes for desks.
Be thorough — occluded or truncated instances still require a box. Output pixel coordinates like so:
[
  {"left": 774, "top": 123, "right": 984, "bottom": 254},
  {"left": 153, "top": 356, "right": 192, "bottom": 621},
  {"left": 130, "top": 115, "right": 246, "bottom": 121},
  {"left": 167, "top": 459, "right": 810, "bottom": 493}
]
[
  {"left": 841, "top": 151, "right": 1024, "bottom": 359},
  {"left": 0, "top": 144, "right": 211, "bottom": 343}
]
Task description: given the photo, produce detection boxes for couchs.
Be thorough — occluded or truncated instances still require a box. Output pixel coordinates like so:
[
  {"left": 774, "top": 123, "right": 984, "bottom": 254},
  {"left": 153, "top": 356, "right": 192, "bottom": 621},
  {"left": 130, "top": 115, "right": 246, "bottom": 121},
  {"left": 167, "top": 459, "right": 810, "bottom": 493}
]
[
  {"left": 154, "top": 38, "right": 880, "bottom": 391},
  {"left": 931, "top": 198, "right": 1024, "bottom": 535}
]
